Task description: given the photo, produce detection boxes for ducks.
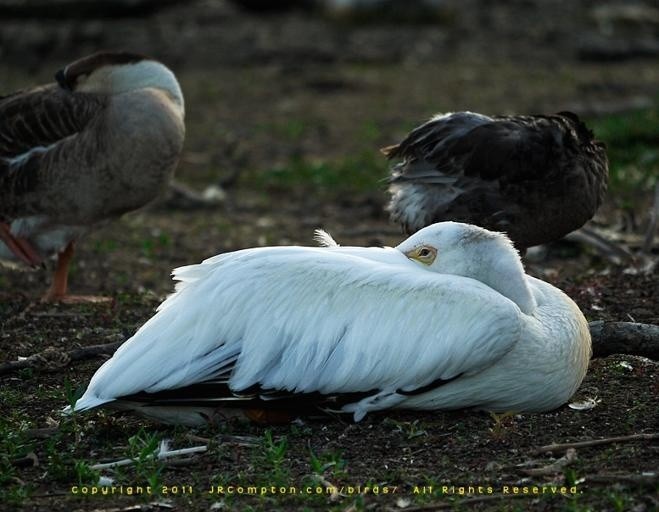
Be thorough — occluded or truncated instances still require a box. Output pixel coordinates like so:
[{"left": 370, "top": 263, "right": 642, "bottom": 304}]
[
  {"left": 374, "top": 109, "right": 610, "bottom": 258},
  {"left": 2, "top": 49, "right": 186, "bottom": 307},
  {"left": 60, "top": 220, "right": 594, "bottom": 423}
]
